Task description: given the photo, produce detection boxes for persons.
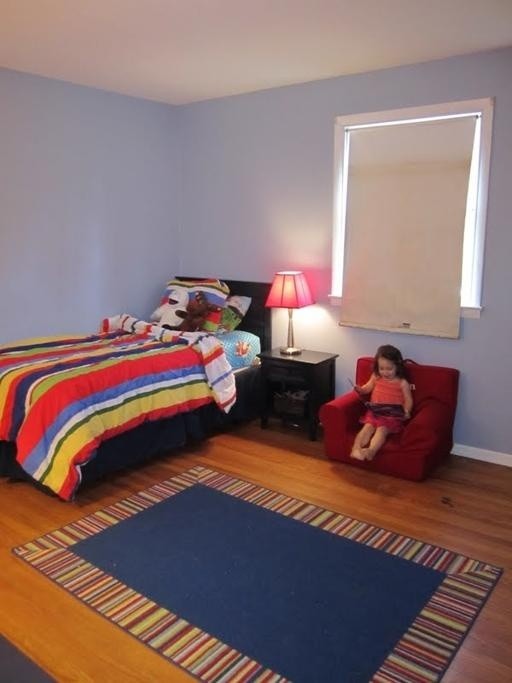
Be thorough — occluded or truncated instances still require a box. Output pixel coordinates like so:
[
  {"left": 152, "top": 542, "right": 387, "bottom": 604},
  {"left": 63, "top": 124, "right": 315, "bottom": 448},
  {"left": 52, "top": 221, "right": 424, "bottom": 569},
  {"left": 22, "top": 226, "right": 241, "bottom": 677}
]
[{"left": 350, "top": 345, "right": 413, "bottom": 461}]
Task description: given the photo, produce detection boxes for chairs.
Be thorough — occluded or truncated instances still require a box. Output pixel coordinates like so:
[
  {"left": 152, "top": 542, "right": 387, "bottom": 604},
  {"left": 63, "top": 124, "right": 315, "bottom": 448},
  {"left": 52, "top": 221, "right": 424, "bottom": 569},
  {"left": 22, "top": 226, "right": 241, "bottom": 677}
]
[{"left": 318, "top": 357, "right": 459, "bottom": 484}]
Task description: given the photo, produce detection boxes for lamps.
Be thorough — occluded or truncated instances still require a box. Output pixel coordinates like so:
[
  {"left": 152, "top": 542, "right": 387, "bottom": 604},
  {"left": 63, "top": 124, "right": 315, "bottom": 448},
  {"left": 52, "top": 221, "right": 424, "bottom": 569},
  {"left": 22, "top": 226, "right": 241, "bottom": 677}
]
[{"left": 265, "top": 270, "right": 316, "bottom": 355}]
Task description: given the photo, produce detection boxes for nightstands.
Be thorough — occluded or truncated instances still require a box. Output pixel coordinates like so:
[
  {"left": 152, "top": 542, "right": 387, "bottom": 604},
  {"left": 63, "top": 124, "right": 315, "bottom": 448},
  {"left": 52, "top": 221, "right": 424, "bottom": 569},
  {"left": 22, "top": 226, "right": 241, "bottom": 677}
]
[{"left": 255, "top": 347, "right": 340, "bottom": 443}]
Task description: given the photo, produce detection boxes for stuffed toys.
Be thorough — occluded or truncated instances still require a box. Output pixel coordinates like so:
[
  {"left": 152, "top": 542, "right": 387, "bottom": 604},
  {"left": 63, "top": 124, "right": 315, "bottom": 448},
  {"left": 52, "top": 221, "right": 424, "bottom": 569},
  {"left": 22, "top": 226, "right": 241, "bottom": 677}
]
[
  {"left": 162, "top": 291, "right": 223, "bottom": 332},
  {"left": 151, "top": 289, "right": 189, "bottom": 327}
]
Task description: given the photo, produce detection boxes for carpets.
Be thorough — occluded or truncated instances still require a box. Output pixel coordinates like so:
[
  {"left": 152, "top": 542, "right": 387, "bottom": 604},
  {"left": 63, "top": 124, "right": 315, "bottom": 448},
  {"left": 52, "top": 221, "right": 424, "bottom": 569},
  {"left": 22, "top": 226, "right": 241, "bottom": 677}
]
[{"left": 11, "top": 465, "right": 503, "bottom": 683}]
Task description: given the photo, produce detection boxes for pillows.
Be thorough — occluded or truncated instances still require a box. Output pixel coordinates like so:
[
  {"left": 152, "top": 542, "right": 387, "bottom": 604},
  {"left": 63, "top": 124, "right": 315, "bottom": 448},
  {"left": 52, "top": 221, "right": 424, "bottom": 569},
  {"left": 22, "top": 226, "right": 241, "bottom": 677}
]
[
  {"left": 150, "top": 279, "right": 229, "bottom": 334},
  {"left": 220, "top": 292, "right": 252, "bottom": 332}
]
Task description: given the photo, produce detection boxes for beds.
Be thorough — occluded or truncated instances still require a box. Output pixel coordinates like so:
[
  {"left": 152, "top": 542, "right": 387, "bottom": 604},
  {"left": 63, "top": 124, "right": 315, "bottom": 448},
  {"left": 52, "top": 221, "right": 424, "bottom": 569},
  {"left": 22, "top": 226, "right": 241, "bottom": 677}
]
[{"left": 0, "top": 276, "right": 272, "bottom": 503}]
